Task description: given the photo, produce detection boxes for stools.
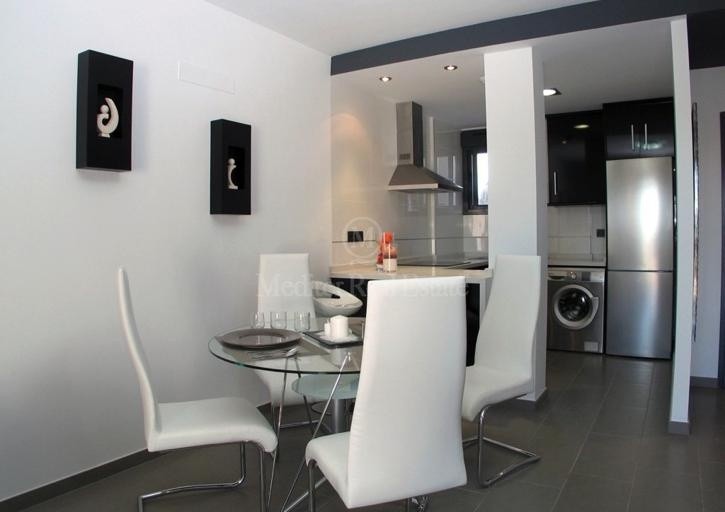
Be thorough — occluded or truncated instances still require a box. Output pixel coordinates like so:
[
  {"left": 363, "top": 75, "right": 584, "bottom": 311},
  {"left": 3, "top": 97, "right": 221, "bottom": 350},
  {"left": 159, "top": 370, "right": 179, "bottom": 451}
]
[{"left": 309, "top": 281, "right": 362, "bottom": 415}]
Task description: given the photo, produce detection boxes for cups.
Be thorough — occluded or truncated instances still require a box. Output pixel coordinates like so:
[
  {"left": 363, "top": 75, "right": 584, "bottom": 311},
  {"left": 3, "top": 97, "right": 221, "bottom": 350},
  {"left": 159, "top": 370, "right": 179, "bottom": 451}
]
[
  {"left": 270, "top": 312, "right": 286, "bottom": 329},
  {"left": 295, "top": 311, "right": 310, "bottom": 333},
  {"left": 250, "top": 311, "right": 265, "bottom": 329},
  {"left": 330, "top": 314, "right": 348, "bottom": 339}
]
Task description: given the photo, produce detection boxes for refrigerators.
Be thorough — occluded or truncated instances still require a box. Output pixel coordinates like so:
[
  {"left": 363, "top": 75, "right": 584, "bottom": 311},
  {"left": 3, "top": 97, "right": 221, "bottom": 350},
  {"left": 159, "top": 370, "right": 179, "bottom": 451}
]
[{"left": 605, "top": 156, "right": 674, "bottom": 361}]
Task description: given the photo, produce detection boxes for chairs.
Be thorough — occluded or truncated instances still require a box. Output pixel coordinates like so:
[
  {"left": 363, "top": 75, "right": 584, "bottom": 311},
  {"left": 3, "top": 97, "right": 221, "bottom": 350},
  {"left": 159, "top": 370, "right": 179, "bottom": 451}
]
[
  {"left": 304, "top": 275, "right": 468, "bottom": 511},
  {"left": 116, "top": 267, "right": 279, "bottom": 511},
  {"left": 458, "top": 254, "right": 547, "bottom": 489},
  {"left": 254, "top": 251, "right": 331, "bottom": 443}
]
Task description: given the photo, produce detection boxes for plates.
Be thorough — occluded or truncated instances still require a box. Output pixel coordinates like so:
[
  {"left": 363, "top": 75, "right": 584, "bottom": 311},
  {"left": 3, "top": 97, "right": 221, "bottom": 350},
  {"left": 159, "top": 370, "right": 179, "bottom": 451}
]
[
  {"left": 222, "top": 327, "right": 300, "bottom": 350},
  {"left": 303, "top": 328, "right": 363, "bottom": 348}
]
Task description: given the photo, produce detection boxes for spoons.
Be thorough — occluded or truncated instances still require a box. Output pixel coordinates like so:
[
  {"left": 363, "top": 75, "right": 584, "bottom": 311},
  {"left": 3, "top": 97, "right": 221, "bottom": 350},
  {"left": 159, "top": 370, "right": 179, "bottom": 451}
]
[{"left": 252, "top": 347, "right": 300, "bottom": 360}]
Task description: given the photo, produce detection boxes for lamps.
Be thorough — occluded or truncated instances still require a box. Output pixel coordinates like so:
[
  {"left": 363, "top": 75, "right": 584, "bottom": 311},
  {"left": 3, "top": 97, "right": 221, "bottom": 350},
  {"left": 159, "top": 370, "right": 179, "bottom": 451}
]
[
  {"left": 542, "top": 88, "right": 561, "bottom": 97},
  {"left": 443, "top": 65, "right": 458, "bottom": 72},
  {"left": 379, "top": 76, "right": 391, "bottom": 83}
]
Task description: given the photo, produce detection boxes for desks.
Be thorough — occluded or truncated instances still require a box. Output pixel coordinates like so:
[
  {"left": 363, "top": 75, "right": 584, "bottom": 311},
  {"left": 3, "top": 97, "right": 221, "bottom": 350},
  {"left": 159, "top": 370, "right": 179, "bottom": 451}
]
[{"left": 331, "top": 261, "right": 484, "bottom": 366}]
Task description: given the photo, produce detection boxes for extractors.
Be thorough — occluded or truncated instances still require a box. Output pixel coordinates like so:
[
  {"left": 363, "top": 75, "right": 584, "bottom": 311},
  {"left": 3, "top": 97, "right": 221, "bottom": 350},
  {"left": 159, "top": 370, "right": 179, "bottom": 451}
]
[{"left": 386, "top": 101, "right": 464, "bottom": 194}]
